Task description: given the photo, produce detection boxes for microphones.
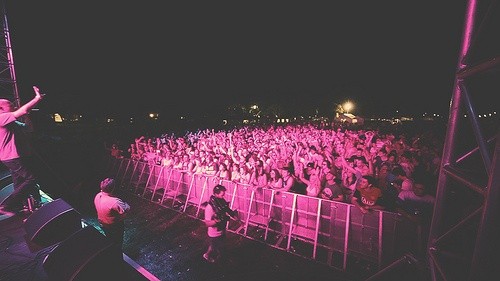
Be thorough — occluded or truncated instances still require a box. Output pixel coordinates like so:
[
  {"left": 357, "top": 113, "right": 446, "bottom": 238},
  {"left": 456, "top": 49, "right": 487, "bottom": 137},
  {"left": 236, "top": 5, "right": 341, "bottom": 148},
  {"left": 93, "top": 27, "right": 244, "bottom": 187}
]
[{"left": 29, "top": 108, "right": 40, "bottom": 112}]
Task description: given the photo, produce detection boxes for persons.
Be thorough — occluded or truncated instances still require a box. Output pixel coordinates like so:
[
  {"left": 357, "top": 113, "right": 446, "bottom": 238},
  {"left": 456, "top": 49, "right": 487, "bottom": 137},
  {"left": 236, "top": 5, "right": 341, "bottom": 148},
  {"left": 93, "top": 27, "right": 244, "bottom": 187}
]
[
  {"left": 202, "top": 184, "right": 241, "bottom": 263},
  {"left": 0, "top": 84, "right": 45, "bottom": 215},
  {"left": 94, "top": 177, "right": 131, "bottom": 277},
  {"left": 111, "top": 121, "right": 445, "bottom": 213}
]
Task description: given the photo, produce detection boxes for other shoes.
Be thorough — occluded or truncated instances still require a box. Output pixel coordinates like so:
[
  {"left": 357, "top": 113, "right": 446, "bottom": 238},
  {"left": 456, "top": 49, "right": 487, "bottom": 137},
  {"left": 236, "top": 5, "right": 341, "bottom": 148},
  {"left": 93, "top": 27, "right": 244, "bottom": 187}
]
[{"left": 202, "top": 254, "right": 216, "bottom": 263}]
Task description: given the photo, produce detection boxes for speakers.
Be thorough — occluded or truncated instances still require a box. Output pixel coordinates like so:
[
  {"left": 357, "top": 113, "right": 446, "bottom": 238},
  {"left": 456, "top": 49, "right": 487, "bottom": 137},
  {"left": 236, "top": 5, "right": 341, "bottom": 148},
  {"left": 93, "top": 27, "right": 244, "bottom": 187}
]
[
  {"left": 42, "top": 222, "right": 116, "bottom": 281},
  {"left": 0, "top": 169, "right": 41, "bottom": 209},
  {"left": 22, "top": 198, "right": 82, "bottom": 247}
]
